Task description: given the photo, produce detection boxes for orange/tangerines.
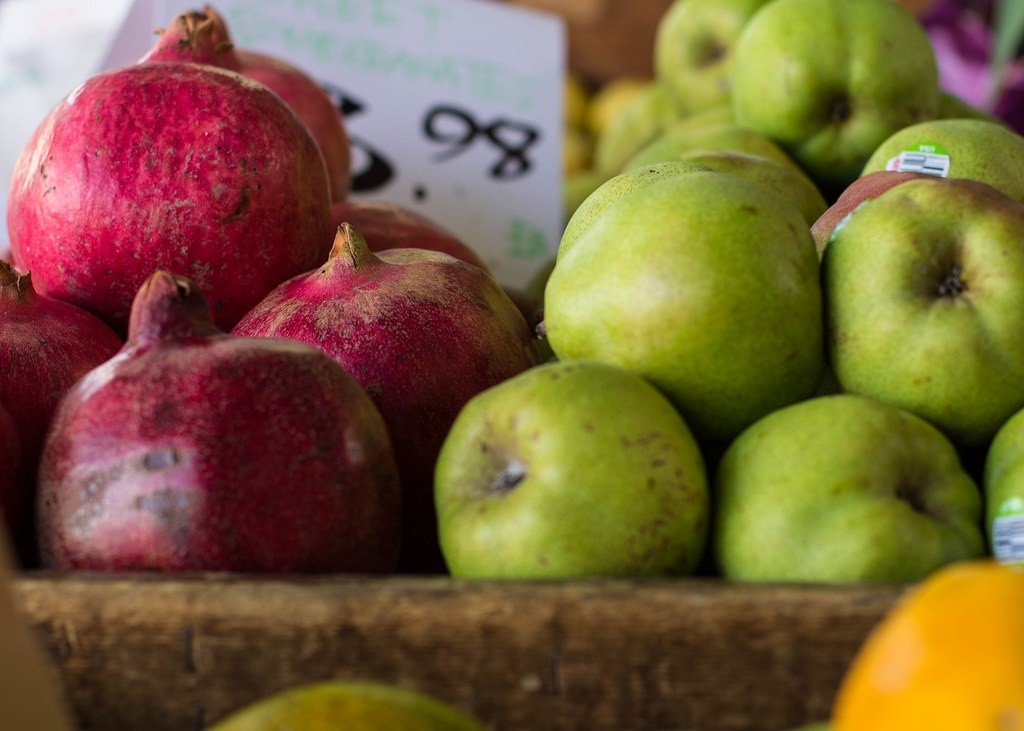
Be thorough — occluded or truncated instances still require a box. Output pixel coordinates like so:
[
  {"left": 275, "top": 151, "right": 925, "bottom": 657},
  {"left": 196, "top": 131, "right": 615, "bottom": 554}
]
[{"left": 0, "top": 553, "right": 1024, "bottom": 730}]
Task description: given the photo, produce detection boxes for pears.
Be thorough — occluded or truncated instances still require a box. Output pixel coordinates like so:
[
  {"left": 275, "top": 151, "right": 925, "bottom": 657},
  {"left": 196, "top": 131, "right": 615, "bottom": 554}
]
[{"left": 433, "top": 0, "right": 1024, "bottom": 601}]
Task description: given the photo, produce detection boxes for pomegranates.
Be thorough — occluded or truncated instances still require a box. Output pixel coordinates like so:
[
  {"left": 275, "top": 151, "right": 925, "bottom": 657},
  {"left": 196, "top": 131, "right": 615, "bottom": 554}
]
[{"left": 0, "top": 2, "right": 536, "bottom": 582}]
